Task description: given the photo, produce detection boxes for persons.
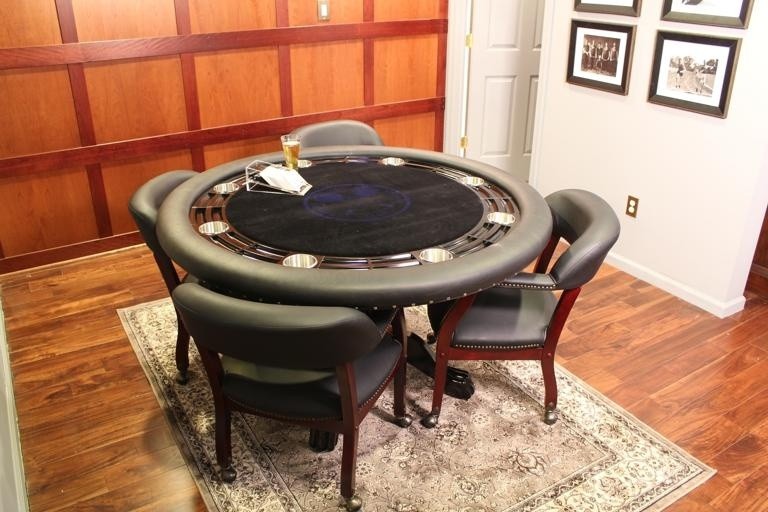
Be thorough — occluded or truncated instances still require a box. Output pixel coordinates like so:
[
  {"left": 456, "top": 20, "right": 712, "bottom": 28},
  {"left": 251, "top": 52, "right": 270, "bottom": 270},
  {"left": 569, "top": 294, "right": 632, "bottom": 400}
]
[
  {"left": 581, "top": 38, "right": 617, "bottom": 75},
  {"left": 668, "top": 56, "right": 716, "bottom": 95}
]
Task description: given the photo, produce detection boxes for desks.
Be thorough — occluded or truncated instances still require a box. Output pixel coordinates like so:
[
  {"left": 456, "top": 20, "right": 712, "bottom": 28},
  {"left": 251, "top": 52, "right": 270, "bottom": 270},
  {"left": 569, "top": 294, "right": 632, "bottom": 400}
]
[{"left": 156, "top": 146, "right": 553, "bottom": 453}]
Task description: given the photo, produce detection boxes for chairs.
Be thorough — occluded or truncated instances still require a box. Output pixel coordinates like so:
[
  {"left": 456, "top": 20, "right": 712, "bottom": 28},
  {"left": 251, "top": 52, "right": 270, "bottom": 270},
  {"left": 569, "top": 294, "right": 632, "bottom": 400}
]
[
  {"left": 423, "top": 189, "right": 620, "bottom": 428},
  {"left": 289, "top": 119, "right": 385, "bottom": 148},
  {"left": 127, "top": 170, "right": 274, "bottom": 384},
  {"left": 172, "top": 283, "right": 414, "bottom": 512}
]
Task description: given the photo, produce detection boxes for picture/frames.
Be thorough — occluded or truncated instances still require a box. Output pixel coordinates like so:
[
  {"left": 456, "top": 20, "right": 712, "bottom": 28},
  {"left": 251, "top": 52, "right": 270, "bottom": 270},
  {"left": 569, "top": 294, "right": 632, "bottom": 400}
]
[
  {"left": 660, "top": 0, "right": 755, "bottom": 30},
  {"left": 573, "top": 0, "right": 643, "bottom": 17},
  {"left": 566, "top": 18, "right": 637, "bottom": 96},
  {"left": 647, "top": 30, "right": 743, "bottom": 119}
]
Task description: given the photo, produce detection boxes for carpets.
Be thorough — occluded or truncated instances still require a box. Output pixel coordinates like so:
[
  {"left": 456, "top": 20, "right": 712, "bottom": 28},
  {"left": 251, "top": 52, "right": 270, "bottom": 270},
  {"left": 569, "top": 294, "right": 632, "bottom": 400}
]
[{"left": 116, "top": 296, "right": 718, "bottom": 512}]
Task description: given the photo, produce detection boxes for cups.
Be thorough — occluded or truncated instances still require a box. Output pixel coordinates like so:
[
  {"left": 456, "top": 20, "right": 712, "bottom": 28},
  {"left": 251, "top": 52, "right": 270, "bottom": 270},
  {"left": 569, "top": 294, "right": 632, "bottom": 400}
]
[{"left": 279, "top": 134, "right": 301, "bottom": 174}]
